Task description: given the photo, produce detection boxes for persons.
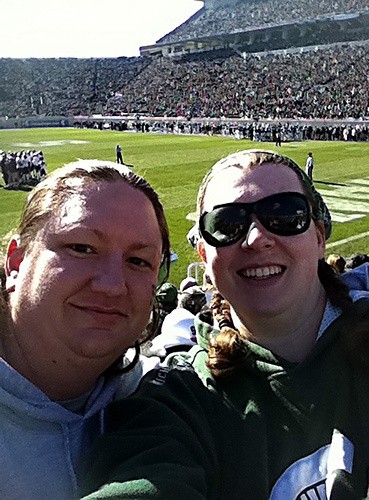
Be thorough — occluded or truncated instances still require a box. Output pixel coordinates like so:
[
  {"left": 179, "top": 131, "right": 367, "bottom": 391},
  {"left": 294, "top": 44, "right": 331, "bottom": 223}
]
[
  {"left": 0, "top": 148, "right": 48, "bottom": 190},
  {"left": 306, "top": 151, "right": 314, "bottom": 182},
  {"left": 72, "top": 148, "right": 369, "bottom": 500},
  {"left": 0, "top": 157, "right": 234, "bottom": 500},
  {"left": 138, "top": 252, "right": 369, "bottom": 352},
  {"left": 116, "top": 145, "right": 125, "bottom": 165},
  {"left": 0, "top": 0, "right": 369, "bottom": 146}
]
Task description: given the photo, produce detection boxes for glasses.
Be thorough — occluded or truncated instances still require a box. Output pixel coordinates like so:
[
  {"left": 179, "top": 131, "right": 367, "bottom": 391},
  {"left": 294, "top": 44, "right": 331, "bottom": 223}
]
[{"left": 198, "top": 191, "right": 316, "bottom": 248}]
